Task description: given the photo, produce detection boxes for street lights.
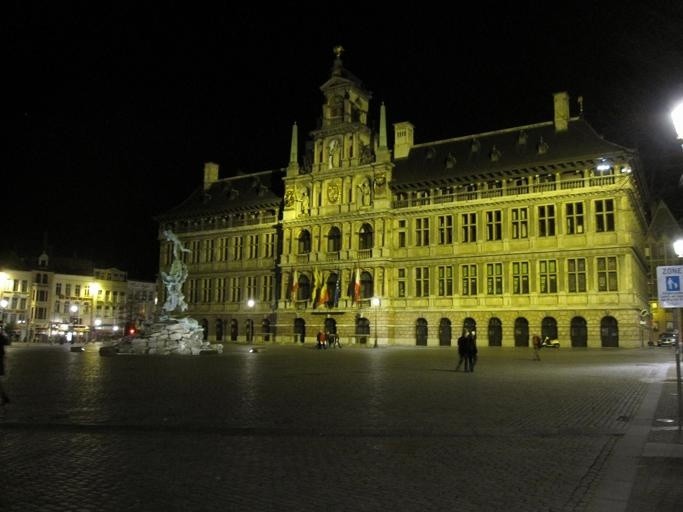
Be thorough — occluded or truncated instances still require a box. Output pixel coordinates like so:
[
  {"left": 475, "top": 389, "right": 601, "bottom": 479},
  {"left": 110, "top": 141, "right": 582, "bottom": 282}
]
[
  {"left": 89, "top": 283, "right": 100, "bottom": 341},
  {"left": 370, "top": 297, "right": 380, "bottom": 349},
  {"left": 246, "top": 299, "right": 256, "bottom": 345},
  {"left": 69, "top": 303, "right": 79, "bottom": 344},
  {"left": 672, "top": 235, "right": 683, "bottom": 351},
  {"left": 0, "top": 298, "right": 9, "bottom": 337}
]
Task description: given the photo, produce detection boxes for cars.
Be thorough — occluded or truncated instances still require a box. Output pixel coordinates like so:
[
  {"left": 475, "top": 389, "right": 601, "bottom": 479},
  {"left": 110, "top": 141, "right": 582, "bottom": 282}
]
[
  {"left": 0, "top": 329, "right": 11, "bottom": 346},
  {"left": 12, "top": 328, "right": 22, "bottom": 336},
  {"left": 73, "top": 324, "right": 91, "bottom": 333},
  {"left": 656, "top": 330, "right": 678, "bottom": 347}
]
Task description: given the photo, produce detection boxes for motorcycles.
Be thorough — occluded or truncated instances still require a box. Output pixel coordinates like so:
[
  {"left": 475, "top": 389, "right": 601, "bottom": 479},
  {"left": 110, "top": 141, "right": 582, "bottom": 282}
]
[{"left": 540, "top": 335, "right": 562, "bottom": 351}]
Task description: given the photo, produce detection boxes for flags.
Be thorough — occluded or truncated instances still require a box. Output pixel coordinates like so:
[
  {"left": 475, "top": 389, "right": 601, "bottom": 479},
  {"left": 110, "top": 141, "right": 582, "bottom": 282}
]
[{"left": 290, "top": 267, "right": 361, "bottom": 307}]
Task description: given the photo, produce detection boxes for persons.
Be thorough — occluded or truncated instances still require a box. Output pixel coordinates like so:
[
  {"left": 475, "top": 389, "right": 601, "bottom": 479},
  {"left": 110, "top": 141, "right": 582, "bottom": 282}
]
[
  {"left": 533, "top": 333, "right": 542, "bottom": 361},
  {"left": 463, "top": 333, "right": 477, "bottom": 372},
  {"left": 456, "top": 332, "right": 468, "bottom": 372},
  {"left": 0, "top": 328, "right": 11, "bottom": 406},
  {"left": 164, "top": 229, "right": 193, "bottom": 260},
  {"left": 318, "top": 330, "right": 342, "bottom": 350}
]
